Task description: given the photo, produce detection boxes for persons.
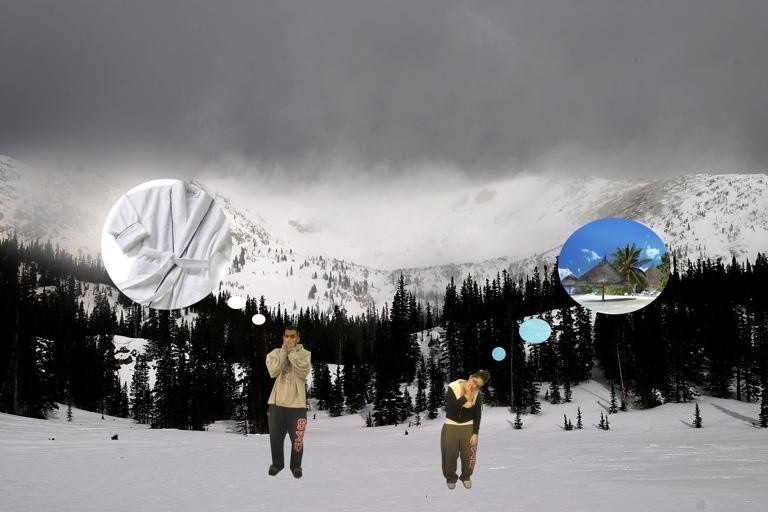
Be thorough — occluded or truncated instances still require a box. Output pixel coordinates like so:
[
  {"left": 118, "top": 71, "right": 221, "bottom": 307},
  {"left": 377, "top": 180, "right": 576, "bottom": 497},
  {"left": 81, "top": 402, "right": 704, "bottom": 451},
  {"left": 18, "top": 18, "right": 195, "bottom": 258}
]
[
  {"left": 441, "top": 369, "right": 492, "bottom": 490},
  {"left": 264, "top": 326, "right": 312, "bottom": 479}
]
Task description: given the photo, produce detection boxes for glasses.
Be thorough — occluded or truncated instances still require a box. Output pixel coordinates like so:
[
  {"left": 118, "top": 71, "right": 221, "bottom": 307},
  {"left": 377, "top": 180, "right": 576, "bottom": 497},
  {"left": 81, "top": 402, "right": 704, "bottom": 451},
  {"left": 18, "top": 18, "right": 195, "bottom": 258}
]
[{"left": 473, "top": 380, "right": 481, "bottom": 391}]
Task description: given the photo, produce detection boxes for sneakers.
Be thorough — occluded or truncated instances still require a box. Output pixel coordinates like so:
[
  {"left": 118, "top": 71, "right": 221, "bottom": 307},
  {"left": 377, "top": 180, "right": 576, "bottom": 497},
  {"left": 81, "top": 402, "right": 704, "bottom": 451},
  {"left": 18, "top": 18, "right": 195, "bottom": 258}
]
[
  {"left": 448, "top": 482, "right": 455, "bottom": 490},
  {"left": 290, "top": 466, "right": 302, "bottom": 478},
  {"left": 464, "top": 480, "right": 472, "bottom": 489},
  {"left": 269, "top": 466, "right": 279, "bottom": 475}
]
